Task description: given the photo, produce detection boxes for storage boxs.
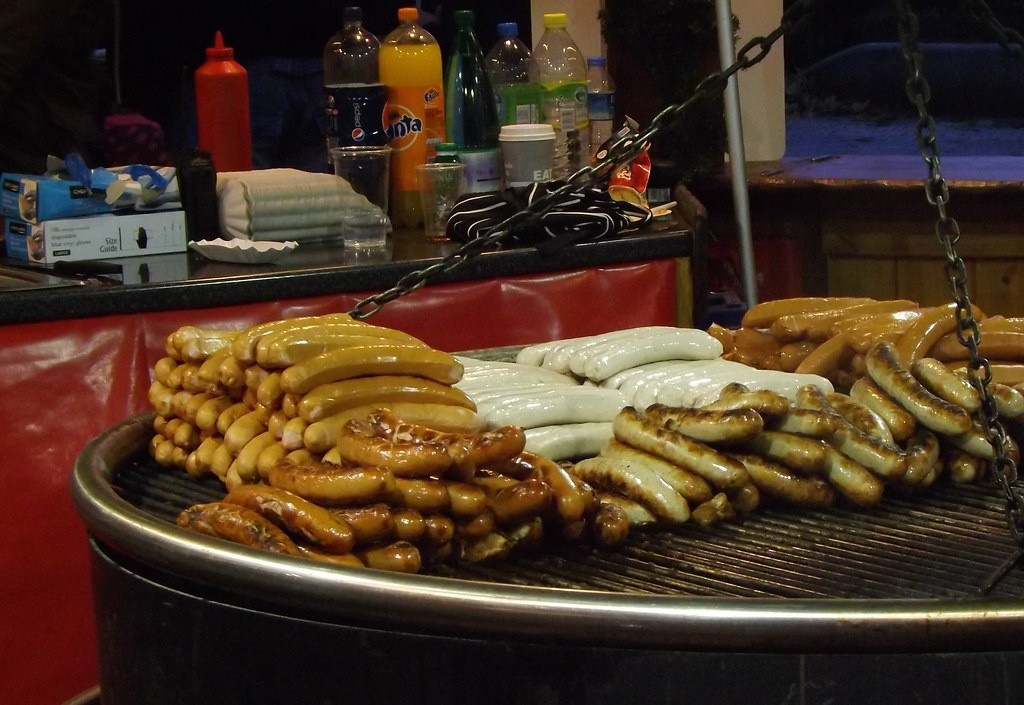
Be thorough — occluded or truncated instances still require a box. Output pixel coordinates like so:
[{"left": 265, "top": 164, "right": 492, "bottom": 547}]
[
  {"left": 0, "top": 165, "right": 187, "bottom": 226},
  {"left": 4, "top": 210, "right": 187, "bottom": 265}
]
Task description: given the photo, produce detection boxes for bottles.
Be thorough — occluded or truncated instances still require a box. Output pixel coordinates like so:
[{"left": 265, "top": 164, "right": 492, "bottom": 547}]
[
  {"left": 322, "top": 0, "right": 616, "bottom": 196},
  {"left": 198, "top": 28, "right": 256, "bottom": 175}
]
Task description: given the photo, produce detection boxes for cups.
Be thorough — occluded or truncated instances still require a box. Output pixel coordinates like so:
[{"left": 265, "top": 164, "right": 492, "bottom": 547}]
[
  {"left": 328, "top": 140, "right": 391, "bottom": 248},
  {"left": 414, "top": 160, "right": 467, "bottom": 242},
  {"left": 497, "top": 122, "right": 557, "bottom": 187}
]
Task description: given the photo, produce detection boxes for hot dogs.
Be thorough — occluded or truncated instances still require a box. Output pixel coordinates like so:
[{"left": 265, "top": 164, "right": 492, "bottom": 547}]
[{"left": 148, "top": 296, "right": 1024, "bottom": 570}]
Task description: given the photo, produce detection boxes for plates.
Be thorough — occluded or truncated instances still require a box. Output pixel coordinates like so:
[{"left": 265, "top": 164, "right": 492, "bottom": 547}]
[{"left": 189, "top": 234, "right": 299, "bottom": 264}]
